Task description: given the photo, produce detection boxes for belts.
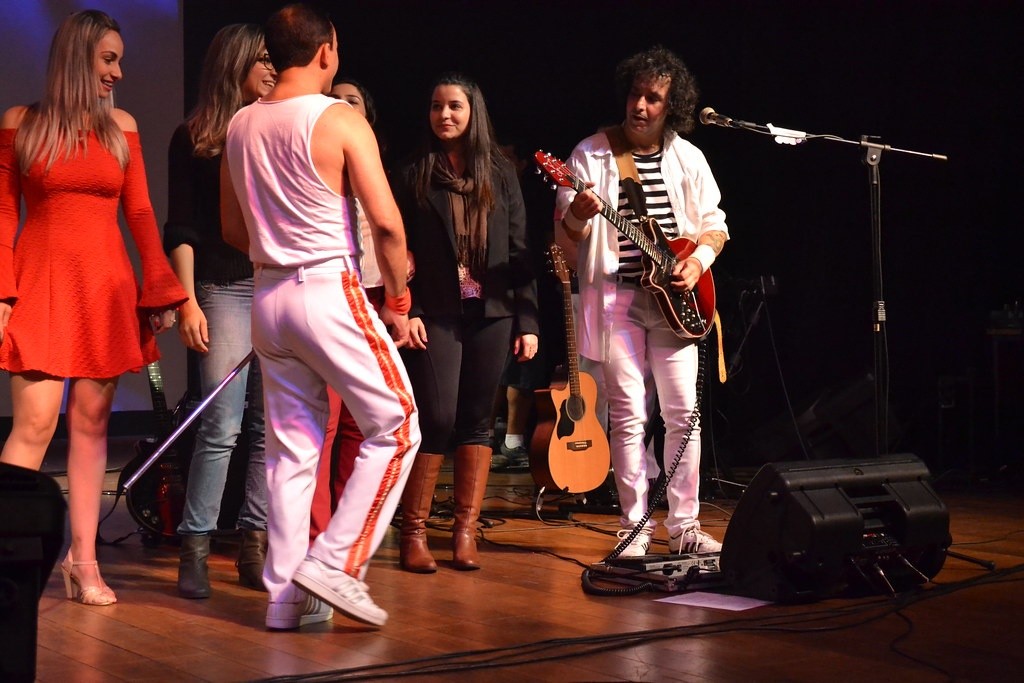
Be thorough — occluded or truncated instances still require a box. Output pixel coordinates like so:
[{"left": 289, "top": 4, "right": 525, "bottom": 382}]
[
  {"left": 252, "top": 266, "right": 347, "bottom": 280},
  {"left": 616, "top": 275, "right": 641, "bottom": 286}
]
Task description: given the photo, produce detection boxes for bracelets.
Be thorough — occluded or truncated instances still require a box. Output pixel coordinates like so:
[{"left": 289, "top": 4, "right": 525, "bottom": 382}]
[
  {"left": 563, "top": 207, "right": 588, "bottom": 231},
  {"left": 688, "top": 245, "right": 717, "bottom": 274},
  {"left": 385, "top": 287, "right": 411, "bottom": 314}
]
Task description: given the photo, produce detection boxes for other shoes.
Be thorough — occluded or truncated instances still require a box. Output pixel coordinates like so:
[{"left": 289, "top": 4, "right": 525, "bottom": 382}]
[
  {"left": 645, "top": 477, "right": 668, "bottom": 505},
  {"left": 499, "top": 439, "right": 529, "bottom": 460},
  {"left": 598, "top": 477, "right": 620, "bottom": 505}
]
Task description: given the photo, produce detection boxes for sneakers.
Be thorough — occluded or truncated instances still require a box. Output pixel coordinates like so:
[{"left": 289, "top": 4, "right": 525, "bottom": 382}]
[
  {"left": 613, "top": 526, "right": 655, "bottom": 558},
  {"left": 668, "top": 525, "right": 721, "bottom": 554},
  {"left": 263, "top": 589, "right": 334, "bottom": 629},
  {"left": 290, "top": 557, "right": 388, "bottom": 627}
]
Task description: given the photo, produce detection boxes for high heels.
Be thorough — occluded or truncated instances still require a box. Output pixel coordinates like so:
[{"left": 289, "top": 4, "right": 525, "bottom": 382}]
[{"left": 60, "top": 546, "right": 117, "bottom": 607}]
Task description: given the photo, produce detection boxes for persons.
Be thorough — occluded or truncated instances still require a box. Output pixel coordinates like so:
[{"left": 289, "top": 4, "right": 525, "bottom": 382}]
[
  {"left": 309, "top": 77, "right": 386, "bottom": 544},
  {"left": 163, "top": 23, "right": 277, "bottom": 596},
  {"left": 220, "top": 2, "right": 422, "bottom": 629},
  {"left": 557, "top": 48, "right": 730, "bottom": 556},
  {"left": 0, "top": 9, "right": 190, "bottom": 605},
  {"left": 389, "top": 68, "right": 539, "bottom": 574}
]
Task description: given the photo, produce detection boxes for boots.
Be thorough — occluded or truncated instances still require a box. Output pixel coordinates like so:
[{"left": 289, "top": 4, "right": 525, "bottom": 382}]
[
  {"left": 236, "top": 529, "right": 267, "bottom": 591},
  {"left": 451, "top": 444, "right": 492, "bottom": 569},
  {"left": 178, "top": 534, "right": 210, "bottom": 598},
  {"left": 399, "top": 452, "right": 445, "bottom": 572}
]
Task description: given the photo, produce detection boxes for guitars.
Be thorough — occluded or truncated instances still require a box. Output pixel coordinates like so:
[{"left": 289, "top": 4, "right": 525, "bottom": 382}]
[
  {"left": 528, "top": 145, "right": 718, "bottom": 343},
  {"left": 127, "top": 359, "right": 192, "bottom": 540},
  {"left": 530, "top": 242, "right": 613, "bottom": 494}
]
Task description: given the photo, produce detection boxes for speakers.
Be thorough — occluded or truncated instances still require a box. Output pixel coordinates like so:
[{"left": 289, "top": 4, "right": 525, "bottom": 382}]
[{"left": 720, "top": 453, "right": 951, "bottom": 602}]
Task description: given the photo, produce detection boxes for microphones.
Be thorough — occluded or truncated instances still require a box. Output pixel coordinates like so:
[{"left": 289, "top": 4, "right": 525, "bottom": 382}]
[{"left": 699, "top": 107, "right": 740, "bottom": 127}]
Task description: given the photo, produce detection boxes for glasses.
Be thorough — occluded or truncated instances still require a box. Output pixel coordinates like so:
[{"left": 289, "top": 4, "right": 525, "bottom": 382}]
[{"left": 251, "top": 53, "right": 273, "bottom": 71}]
